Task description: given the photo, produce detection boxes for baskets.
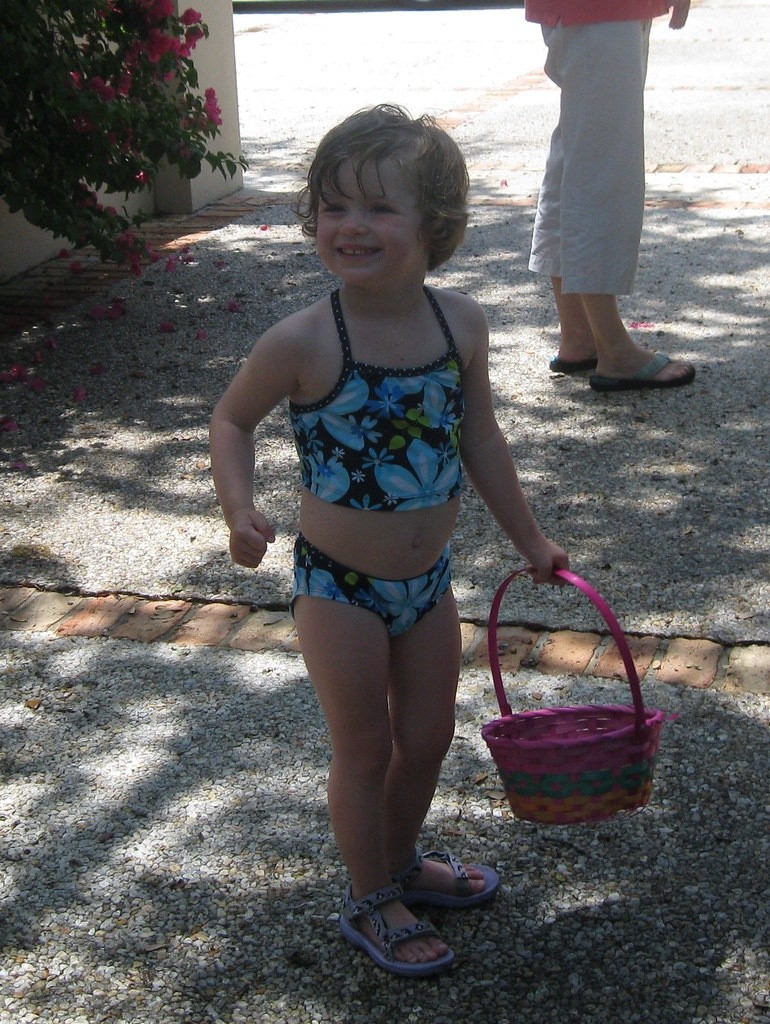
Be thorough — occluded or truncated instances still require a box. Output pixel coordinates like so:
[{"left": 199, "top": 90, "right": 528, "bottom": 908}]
[{"left": 481, "top": 567, "right": 663, "bottom": 823}]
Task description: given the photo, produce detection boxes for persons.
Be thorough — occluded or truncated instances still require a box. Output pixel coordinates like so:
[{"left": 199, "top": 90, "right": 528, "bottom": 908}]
[
  {"left": 210, "top": 104, "right": 571, "bottom": 976},
  {"left": 526, "top": 0, "right": 696, "bottom": 387}
]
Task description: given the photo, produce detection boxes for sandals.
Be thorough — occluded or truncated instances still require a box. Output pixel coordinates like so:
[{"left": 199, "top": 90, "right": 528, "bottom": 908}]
[
  {"left": 387, "top": 848, "right": 500, "bottom": 908},
  {"left": 338, "top": 881, "right": 455, "bottom": 977}
]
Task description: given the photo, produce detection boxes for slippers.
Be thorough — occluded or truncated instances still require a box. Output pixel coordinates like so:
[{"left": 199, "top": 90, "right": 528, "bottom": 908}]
[
  {"left": 588, "top": 351, "right": 696, "bottom": 391},
  {"left": 548, "top": 352, "right": 597, "bottom": 374}
]
